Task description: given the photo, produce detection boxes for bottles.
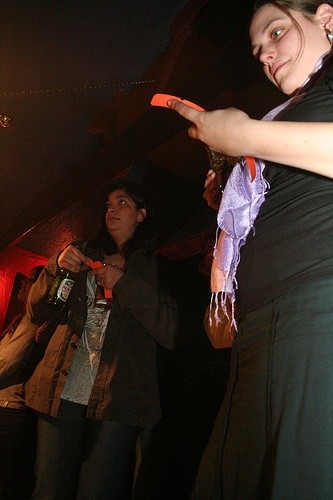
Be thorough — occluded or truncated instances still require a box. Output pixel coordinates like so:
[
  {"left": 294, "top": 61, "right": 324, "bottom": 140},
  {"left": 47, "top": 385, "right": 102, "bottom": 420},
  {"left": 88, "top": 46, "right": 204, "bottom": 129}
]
[{"left": 43, "top": 242, "right": 81, "bottom": 308}]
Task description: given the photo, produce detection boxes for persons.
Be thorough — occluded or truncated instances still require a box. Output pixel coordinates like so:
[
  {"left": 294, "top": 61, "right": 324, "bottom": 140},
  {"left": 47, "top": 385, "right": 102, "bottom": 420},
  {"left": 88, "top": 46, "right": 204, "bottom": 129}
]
[
  {"left": 166, "top": 0, "right": 333, "bottom": 500},
  {"left": 0, "top": 181, "right": 166, "bottom": 500}
]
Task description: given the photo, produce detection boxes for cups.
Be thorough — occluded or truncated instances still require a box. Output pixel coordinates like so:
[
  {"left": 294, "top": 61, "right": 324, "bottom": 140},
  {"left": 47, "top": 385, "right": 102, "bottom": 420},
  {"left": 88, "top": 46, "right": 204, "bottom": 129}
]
[
  {"left": 207, "top": 147, "right": 236, "bottom": 202},
  {"left": 95, "top": 262, "right": 115, "bottom": 308}
]
